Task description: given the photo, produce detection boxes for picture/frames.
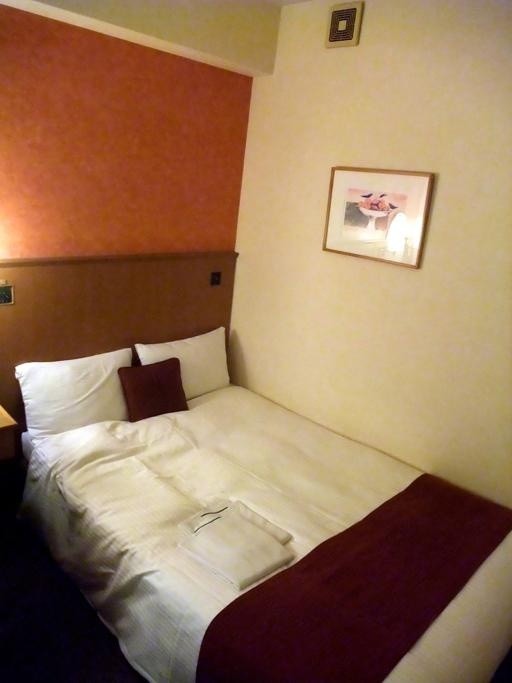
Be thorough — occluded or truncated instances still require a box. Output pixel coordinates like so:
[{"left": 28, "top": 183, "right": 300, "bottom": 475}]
[{"left": 321, "top": 167, "right": 435, "bottom": 269}]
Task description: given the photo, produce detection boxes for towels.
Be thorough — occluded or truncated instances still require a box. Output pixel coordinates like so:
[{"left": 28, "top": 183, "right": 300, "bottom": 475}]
[{"left": 175, "top": 496, "right": 297, "bottom": 591}]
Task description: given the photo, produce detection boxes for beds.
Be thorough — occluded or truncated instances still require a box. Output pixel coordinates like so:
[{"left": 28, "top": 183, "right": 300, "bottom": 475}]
[{"left": 1, "top": 251, "right": 512, "bottom": 683}]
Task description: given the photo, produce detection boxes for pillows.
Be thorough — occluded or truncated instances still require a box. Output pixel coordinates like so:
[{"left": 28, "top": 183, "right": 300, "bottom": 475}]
[
  {"left": 14, "top": 349, "right": 137, "bottom": 432},
  {"left": 133, "top": 325, "right": 232, "bottom": 414},
  {"left": 117, "top": 357, "right": 189, "bottom": 423}
]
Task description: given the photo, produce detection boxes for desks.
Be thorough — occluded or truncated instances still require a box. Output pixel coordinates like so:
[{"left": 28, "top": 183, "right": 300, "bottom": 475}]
[{"left": 2, "top": 390, "right": 18, "bottom": 459}]
